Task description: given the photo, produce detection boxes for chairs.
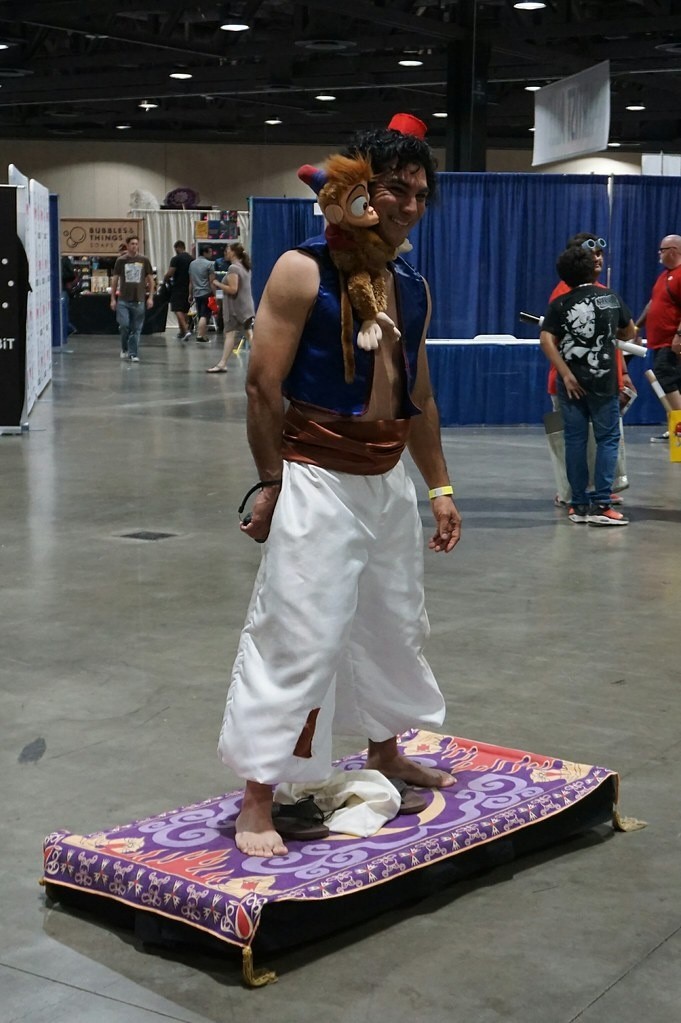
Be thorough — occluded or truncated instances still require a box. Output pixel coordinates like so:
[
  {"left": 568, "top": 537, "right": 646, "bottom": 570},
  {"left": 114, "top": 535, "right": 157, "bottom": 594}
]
[{"left": 473, "top": 334, "right": 517, "bottom": 339}]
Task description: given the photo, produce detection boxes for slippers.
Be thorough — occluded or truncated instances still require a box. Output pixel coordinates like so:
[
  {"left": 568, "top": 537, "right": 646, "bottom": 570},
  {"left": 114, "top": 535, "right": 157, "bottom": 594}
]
[
  {"left": 271, "top": 797, "right": 329, "bottom": 841},
  {"left": 386, "top": 777, "right": 426, "bottom": 814}
]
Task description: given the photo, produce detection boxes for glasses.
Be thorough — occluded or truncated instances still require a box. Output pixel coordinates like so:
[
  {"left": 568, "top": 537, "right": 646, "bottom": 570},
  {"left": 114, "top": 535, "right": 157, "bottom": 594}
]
[
  {"left": 658, "top": 247, "right": 671, "bottom": 253},
  {"left": 581, "top": 238, "right": 607, "bottom": 251}
]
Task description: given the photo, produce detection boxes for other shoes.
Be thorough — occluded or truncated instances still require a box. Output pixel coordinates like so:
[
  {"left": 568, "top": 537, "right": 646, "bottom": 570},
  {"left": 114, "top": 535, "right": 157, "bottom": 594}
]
[
  {"left": 173, "top": 330, "right": 192, "bottom": 342},
  {"left": 68, "top": 327, "right": 77, "bottom": 337},
  {"left": 585, "top": 475, "right": 629, "bottom": 496},
  {"left": 553, "top": 493, "right": 571, "bottom": 509}
]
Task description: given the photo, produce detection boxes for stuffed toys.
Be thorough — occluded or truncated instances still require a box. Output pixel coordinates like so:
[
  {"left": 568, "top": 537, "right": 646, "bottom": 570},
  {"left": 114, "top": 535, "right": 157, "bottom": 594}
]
[{"left": 296, "top": 154, "right": 412, "bottom": 381}]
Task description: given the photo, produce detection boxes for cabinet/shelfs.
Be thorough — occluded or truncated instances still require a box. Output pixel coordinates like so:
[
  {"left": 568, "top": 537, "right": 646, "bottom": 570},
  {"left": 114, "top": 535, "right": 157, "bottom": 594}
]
[
  {"left": 73, "top": 260, "right": 91, "bottom": 294},
  {"left": 194, "top": 239, "right": 242, "bottom": 299}
]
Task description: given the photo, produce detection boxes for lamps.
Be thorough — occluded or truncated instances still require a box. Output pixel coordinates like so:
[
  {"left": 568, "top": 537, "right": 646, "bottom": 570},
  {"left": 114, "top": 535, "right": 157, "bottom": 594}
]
[
  {"left": 115, "top": 121, "right": 134, "bottom": 130},
  {"left": 263, "top": 115, "right": 282, "bottom": 126},
  {"left": 394, "top": 51, "right": 424, "bottom": 69},
  {"left": 314, "top": 91, "right": 337, "bottom": 103},
  {"left": 168, "top": 65, "right": 193, "bottom": 80},
  {"left": 219, "top": 2, "right": 251, "bottom": 33},
  {"left": 513, "top": 0, "right": 547, "bottom": 12},
  {"left": 432, "top": 108, "right": 448, "bottom": 118},
  {"left": 523, "top": 82, "right": 544, "bottom": 92},
  {"left": 624, "top": 101, "right": 648, "bottom": 112},
  {"left": 137, "top": 99, "right": 159, "bottom": 111}
]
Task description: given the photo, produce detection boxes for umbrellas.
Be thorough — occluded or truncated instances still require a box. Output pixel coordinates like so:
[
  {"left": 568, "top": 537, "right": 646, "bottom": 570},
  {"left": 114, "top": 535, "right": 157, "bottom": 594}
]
[{"left": 164, "top": 187, "right": 201, "bottom": 210}]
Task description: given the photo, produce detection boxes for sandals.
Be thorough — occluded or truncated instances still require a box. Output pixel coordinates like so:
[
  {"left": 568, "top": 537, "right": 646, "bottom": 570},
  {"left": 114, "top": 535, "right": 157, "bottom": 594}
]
[{"left": 206, "top": 364, "right": 227, "bottom": 373}]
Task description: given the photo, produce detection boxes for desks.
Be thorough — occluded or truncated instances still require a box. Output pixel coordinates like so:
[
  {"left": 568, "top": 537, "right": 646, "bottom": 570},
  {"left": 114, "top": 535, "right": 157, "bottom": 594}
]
[
  {"left": 72, "top": 292, "right": 169, "bottom": 335},
  {"left": 427, "top": 338, "right": 658, "bottom": 428}
]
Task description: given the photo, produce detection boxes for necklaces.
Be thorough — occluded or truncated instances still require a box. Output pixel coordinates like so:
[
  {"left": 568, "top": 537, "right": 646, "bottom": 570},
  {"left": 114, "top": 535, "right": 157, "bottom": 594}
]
[{"left": 576, "top": 283, "right": 593, "bottom": 287}]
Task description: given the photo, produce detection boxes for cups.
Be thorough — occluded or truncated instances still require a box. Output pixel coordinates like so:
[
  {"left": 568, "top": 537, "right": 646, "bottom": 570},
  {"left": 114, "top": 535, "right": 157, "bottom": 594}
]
[{"left": 619, "top": 385, "right": 638, "bottom": 416}]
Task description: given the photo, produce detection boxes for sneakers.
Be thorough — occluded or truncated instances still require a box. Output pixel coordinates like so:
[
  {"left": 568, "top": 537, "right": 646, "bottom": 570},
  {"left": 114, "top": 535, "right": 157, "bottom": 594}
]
[
  {"left": 650, "top": 431, "right": 669, "bottom": 443},
  {"left": 587, "top": 503, "right": 630, "bottom": 525},
  {"left": 120, "top": 349, "right": 140, "bottom": 362},
  {"left": 568, "top": 507, "right": 588, "bottom": 523},
  {"left": 196, "top": 336, "right": 211, "bottom": 343}
]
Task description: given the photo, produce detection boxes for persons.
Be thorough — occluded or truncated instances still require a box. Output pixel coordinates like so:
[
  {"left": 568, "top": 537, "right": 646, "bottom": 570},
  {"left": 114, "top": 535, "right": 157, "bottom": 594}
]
[
  {"left": 219, "top": 115, "right": 461, "bottom": 857},
  {"left": 635, "top": 234, "right": 681, "bottom": 442},
  {"left": 206, "top": 243, "right": 255, "bottom": 373},
  {"left": 60, "top": 256, "right": 79, "bottom": 338},
  {"left": 118, "top": 243, "right": 127, "bottom": 255},
  {"left": 165, "top": 240, "right": 216, "bottom": 344},
  {"left": 110, "top": 235, "right": 154, "bottom": 362},
  {"left": 540, "top": 233, "right": 653, "bottom": 527}
]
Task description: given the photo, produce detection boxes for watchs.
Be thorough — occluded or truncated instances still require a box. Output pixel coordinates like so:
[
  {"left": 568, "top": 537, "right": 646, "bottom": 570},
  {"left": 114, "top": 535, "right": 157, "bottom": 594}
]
[{"left": 676, "top": 330, "right": 681, "bottom": 337}]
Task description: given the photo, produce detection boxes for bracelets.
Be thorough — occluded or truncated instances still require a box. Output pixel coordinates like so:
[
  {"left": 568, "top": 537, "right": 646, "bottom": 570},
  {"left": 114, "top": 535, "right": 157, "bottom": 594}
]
[
  {"left": 622, "top": 372, "right": 629, "bottom": 375},
  {"left": 149, "top": 293, "right": 154, "bottom": 299},
  {"left": 428, "top": 485, "right": 453, "bottom": 499},
  {"left": 634, "top": 326, "right": 642, "bottom": 332}
]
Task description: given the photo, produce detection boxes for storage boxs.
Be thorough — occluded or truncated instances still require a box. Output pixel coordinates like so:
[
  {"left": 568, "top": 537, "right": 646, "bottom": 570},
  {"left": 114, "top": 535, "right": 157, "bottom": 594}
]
[
  {"left": 222, "top": 209, "right": 239, "bottom": 219},
  {"left": 220, "top": 221, "right": 241, "bottom": 238},
  {"left": 208, "top": 221, "right": 221, "bottom": 239},
  {"left": 192, "top": 220, "right": 208, "bottom": 239}
]
